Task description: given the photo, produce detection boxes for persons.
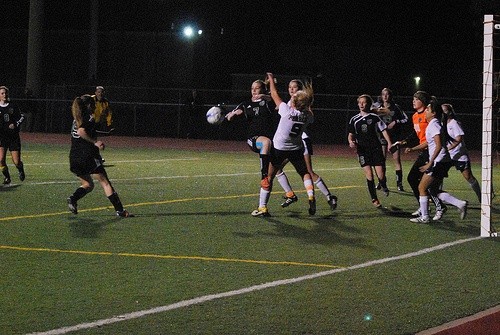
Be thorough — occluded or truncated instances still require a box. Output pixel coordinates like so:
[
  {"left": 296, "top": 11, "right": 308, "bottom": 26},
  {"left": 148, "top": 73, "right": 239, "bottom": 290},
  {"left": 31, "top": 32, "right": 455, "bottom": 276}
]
[
  {"left": 347, "top": 94, "right": 391, "bottom": 208},
  {"left": 66, "top": 94, "right": 134, "bottom": 217},
  {"left": 0, "top": 86, "right": 25, "bottom": 185},
  {"left": 370, "top": 88, "right": 410, "bottom": 192},
  {"left": 90, "top": 86, "right": 116, "bottom": 135},
  {"left": 223, "top": 71, "right": 337, "bottom": 216},
  {"left": 402, "top": 91, "right": 481, "bottom": 224}
]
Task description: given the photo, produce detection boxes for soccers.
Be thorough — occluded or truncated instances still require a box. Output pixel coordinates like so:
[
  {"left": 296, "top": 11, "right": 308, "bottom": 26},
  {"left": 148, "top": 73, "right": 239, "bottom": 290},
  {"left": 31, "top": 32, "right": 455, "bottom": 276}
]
[{"left": 207, "top": 106, "right": 226, "bottom": 124}]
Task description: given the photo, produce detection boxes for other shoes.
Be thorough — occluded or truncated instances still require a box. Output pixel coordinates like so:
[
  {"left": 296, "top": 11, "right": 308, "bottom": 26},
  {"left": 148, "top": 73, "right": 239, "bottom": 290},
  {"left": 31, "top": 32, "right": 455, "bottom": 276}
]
[
  {"left": 380, "top": 186, "right": 390, "bottom": 196},
  {"left": 372, "top": 199, "right": 382, "bottom": 208},
  {"left": 397, "top": 185, "right": 403, "bottom": 191},
  {"left": 375, "top": 176, "right": 386, "bottom": 189}
]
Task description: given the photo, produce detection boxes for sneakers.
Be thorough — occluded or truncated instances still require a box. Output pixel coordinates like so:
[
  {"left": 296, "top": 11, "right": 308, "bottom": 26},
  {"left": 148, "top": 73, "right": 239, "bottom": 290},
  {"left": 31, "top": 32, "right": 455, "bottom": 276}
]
[
  {"left": 281, "top": 194, "right": 298, "bottom": 208},
  {"left": 66, "top": 196, "right": 78, "bottom": 215},
  {"left": 411, "top": 206, "right": 431, "bottom": 216},
  {"left": 19, "top": 170, "right": 25, "bottom": 181},
  {"left": 251, "top": 208, "right": 270, "bottom": 218},
  {"left": 409, "top": 216, "right": 430, "bottom": 224},
  {"left": 327, "top": 196, "right": 337, "bottom": 210},
  {"left": 432, "top": 202, "right": 447, "bottom": 221},
  {"left": 2, "top": 178, "right": 11, "bottom": 184},
  {"left": 116, "top": 208, "right": 135, "bottom": 218},
  {"left": 308, "top": 197, "right": 316, "bottom": 215},
  {"left": 460, "top": 200, "right": 468, "bottom": 220}
]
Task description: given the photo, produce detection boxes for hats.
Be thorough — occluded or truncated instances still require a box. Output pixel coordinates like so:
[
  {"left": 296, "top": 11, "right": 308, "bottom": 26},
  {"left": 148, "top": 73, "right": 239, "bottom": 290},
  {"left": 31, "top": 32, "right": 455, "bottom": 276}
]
[{"left": 413, "top": 90, "right": 429, "bottom": 106}]
